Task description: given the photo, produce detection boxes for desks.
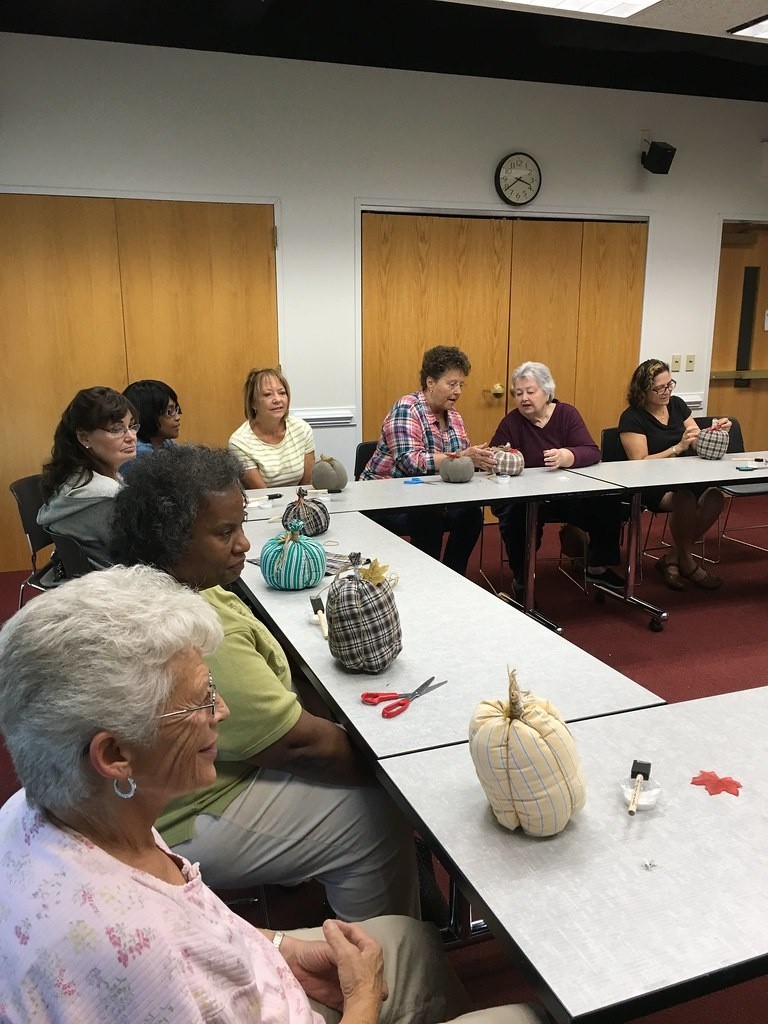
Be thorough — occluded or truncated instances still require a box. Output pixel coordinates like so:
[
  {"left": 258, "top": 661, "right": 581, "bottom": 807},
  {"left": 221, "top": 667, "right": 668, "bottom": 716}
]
[
  {"left": 559, "top": 450, "right": 768, "bottom": 633},
  {"left": 236, "top": 511, "right": 666, "bottom": 765},
  {"left": 376, "top": 686, "right": 768, "bottom": 1024},
  {"left": 242, "top": 466, "right": 626, "bottom": 635}
]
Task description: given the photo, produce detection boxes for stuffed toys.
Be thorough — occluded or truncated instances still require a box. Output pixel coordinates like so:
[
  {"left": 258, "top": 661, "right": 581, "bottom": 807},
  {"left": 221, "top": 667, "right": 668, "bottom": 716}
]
[
  {"left": 696, "top": 419, "right": 729, "bottom": 460},
  {"left": 468, "top": 670, "right": 586, "bottom": 837},
  {"left": 260, "top": 521, "right": 326, "bottom": 590},
  {"left": 312, "top": 458, "right": 348, "bottom": 491},
  {"left": 439, "top": 449, "right": 474, "bottom": 484},
  {"left": 490, "top": 443, "right": 524, "bottom": 476},
  {"left": 328, "top": 551, "right": 403, "bottom": 675},
  {"left": 282, "top": 486, "right": 330, "bottom": 535}
]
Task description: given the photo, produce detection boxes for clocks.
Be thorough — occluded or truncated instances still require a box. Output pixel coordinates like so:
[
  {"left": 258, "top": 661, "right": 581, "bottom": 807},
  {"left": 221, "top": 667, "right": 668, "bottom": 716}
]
[{"left": 494, "top": 152, "right": 541, "bottom": 207}]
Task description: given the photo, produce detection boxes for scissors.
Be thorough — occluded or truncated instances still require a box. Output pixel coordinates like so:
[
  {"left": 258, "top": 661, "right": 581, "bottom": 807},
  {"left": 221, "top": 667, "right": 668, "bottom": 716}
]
[
  {"left": 404, "top": 477, "right": 441, "bottom": 485},
  {"left": 361, "top": 677, "right": 448, "bottom": 719},
  {"left": 736, "top": 466, "right": 768, "bottom": 471}
]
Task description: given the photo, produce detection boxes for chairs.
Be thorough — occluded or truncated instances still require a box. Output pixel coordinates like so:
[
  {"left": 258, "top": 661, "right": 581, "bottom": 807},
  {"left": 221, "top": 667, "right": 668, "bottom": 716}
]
[
  {"left": 355, "top": 440, "right": 379, "bottom": 482},
  {"left": 599, "top": 416, "right": 768, "bottom": 586},
  {"left": 9, "top": 474, "right": 61, "bottom": 611}
]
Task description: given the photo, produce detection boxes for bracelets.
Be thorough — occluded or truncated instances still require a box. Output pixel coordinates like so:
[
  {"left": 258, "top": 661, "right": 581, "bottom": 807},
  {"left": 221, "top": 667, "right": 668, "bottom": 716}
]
[
  {"left": 273, "top": 931, "right": 284, "bottom": 949},
  {"left": 671, "top": 446, "right": 678, "bottom": 456}
]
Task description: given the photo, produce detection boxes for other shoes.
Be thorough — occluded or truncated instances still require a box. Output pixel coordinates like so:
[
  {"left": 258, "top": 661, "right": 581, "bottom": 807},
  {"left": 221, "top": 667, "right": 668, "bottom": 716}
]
[
  {"left": 511, "top": 577, "right": 537, "bottom": 610},
  {"left": 584, "top": 565, "right": 626, "bottom": 587},
  {"left": 656, "top": 554, "right": 691, "bottom": 590},
  {"left": 678, "top": 566, "right": 721, "bottom": 588}
]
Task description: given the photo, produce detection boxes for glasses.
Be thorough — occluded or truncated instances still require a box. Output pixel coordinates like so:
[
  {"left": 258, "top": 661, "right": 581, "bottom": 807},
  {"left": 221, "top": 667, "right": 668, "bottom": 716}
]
[
  {"left": 166, "top": 406, "right": 179, "bottom": 417},
  {"left": 97, "top": 424, "right": 140, "bottom": 436},
  {"left": 653, "top": 379, "right": 676, "bottom": 395},
  {"left": 83, "top": 670, "right": 216, "bottom": 756},
  {"left": 436, "top": 378, "right": 466, "bottom": 390}
]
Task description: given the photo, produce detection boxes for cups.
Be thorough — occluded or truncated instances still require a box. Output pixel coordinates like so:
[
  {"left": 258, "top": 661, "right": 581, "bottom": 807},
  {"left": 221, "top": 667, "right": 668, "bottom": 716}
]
[
  {"left": 305, "top": 608, "right": 321, "bottom": 624},
  {"left": 747, "top": 460, "right": 760, "bottom": 468},
  {"left": 259, "top": 497, "right": 283, "bottom": 509},
  {"left": 497, "top": 475, "right": 510, "bottom": 484},
  {"left": 620, "top": 775, "right": 662, "bottom": 810},
  {"left": 318, "top": 492, "right": 332, "bottom": 502}
]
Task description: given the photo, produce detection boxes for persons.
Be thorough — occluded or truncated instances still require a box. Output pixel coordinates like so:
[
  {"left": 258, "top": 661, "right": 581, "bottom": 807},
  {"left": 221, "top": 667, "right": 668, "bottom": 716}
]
[
  {"left": 37, "top": 386, "right": 140, "bottom": 569},
  {"left": 488, "top": 361, "right": 627, "bottom": 609},
  {"left": 1, "top": 562, "right": 553, "bottom": 1024},
  {"left": 118, "top": 380, "right": 182, "bottom": 483},
  {"left": 108, "top": 441, "right": 403, "bottom": 922},
  {"left": 360, "top": 344, "right": 498, "bottom": 576},
  {"left": 228, "top": 368, "right": 315, "bottom": 488},
  {"left": 616, "top": 359, "right": 732, "bottom": 589}
]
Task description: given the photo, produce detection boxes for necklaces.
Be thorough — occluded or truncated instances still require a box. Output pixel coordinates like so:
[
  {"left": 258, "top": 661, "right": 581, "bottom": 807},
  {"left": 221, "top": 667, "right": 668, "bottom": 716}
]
[{"left": 661, "top": 410, "right": 665, "bottom": 419}]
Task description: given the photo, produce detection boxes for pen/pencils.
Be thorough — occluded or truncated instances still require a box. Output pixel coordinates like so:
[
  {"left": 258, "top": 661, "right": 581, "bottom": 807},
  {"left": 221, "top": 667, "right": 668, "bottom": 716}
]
[
  {"left": 731, "top": 457, "right": 764, "bottom": 462},
  {"left": 628, "top": 774, "right": 643, "bottom": 816},
  {"left": 249, "top": 493, "right": 284, "bottom": 503},
  {"left": 307, "top": 489, "right": 344, "bottom": 493},
  {"left": 486, "top": 472, "right": 501, "bottom": 477},
  {"left": 317, "top": 609, "right": 328, "bottom": 640}
]
